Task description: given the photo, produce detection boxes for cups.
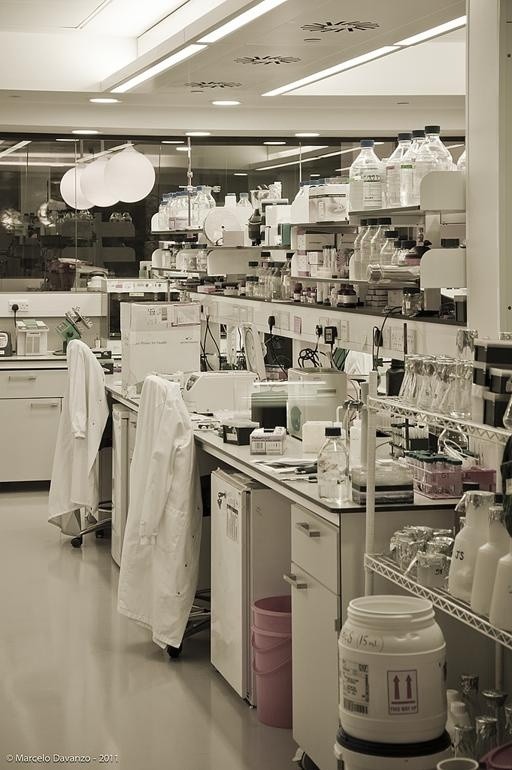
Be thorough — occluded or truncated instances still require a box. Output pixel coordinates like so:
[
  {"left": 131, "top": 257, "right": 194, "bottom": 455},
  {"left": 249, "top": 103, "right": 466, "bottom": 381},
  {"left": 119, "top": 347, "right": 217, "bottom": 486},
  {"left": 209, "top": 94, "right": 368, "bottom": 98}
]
[
  {"left": 416, "top": 552, "right": 446, "bottom": 588},
  {"left": 436, "top": 758, "right": 480, "bottom": 770}
]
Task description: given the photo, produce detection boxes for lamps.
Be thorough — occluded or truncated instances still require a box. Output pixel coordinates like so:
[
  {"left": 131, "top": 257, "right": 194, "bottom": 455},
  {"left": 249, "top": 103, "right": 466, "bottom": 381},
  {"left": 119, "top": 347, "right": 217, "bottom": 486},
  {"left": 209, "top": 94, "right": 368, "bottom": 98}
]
[{"left": 58, "top": 136, "right": 156, "bottom": 211}]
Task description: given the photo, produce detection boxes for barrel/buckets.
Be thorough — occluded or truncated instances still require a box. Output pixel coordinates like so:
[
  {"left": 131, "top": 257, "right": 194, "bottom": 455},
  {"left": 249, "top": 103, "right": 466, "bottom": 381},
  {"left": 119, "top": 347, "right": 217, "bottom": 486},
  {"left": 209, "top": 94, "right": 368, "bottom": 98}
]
[
  {"left": 250, "top": 596, "right": 292, "bottom": 728},
  {"left": 337, "top": 595, "right": 447, "bottom": 743},
  {"left": 333, "top": 728, "right": 454, "bottom": 770}
]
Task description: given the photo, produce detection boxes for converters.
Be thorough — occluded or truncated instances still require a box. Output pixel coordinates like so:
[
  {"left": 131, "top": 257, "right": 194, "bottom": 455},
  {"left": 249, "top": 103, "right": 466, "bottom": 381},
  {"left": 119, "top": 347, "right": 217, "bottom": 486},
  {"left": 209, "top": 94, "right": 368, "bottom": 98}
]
[{"left": 324, "top": 327, "right": 335, "bottom": 344}]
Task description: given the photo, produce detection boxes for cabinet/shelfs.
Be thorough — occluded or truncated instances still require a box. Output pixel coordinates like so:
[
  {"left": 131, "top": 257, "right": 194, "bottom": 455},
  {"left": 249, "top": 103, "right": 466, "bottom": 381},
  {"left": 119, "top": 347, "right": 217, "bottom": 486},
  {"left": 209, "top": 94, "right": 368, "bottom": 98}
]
[{"left": 0, "top": 370, "right": 69, "bottom": 483}]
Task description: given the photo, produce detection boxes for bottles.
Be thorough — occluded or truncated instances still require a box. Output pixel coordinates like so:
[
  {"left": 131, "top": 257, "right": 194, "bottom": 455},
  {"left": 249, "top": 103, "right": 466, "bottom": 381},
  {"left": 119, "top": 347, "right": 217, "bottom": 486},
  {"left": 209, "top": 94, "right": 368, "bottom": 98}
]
[
  {"left": 334, "top": 406, "right": 347, "bottom": 447},
  {"left": 443, "top": 672, "right": 512, "bottom": 760},
  {"left": 316, "top": 426, "right": 349, "bottom": 500},
  {"left": 445, "top": 489, "right": 511, "bottom": 635},
  {"left": 349, "top": 411, "right": 362, "bottom": 469},
  {"left": 391, "top": 421, "right": 464, "bottom": 496},
  {"left": 398, "top": 351, "right": 472, "bottom": 420},
  {"left": 138, "top": 124, "right": 468, "bottom": 324}
]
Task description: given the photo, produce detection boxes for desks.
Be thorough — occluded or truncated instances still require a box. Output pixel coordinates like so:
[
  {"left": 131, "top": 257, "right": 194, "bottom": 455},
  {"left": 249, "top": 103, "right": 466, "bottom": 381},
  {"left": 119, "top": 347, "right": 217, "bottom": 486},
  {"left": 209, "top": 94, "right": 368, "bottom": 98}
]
[{"left": 105, "top": 384, "right": 512, "bottom": 770}]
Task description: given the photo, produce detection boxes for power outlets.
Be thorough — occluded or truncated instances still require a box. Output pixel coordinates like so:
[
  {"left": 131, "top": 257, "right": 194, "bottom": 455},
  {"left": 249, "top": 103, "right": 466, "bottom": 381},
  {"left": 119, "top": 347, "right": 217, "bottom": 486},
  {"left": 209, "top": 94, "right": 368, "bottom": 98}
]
[
  {"left": 201, "top": 301, "right": 414, "bottom": 353},
  {"left": 8, "top": 300, "right": 30, "bottom": 313}
]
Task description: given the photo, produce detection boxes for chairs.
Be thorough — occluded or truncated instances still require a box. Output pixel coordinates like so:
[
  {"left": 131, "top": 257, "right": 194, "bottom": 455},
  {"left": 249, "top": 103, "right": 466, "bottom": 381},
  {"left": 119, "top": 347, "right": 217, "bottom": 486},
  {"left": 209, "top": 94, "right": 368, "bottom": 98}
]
[
  {"left": 66, "top": 338, "right": 112, "bottom": 550},
  {"left": 136, "top": 376, "right": 210, "bottom": 659}
]
